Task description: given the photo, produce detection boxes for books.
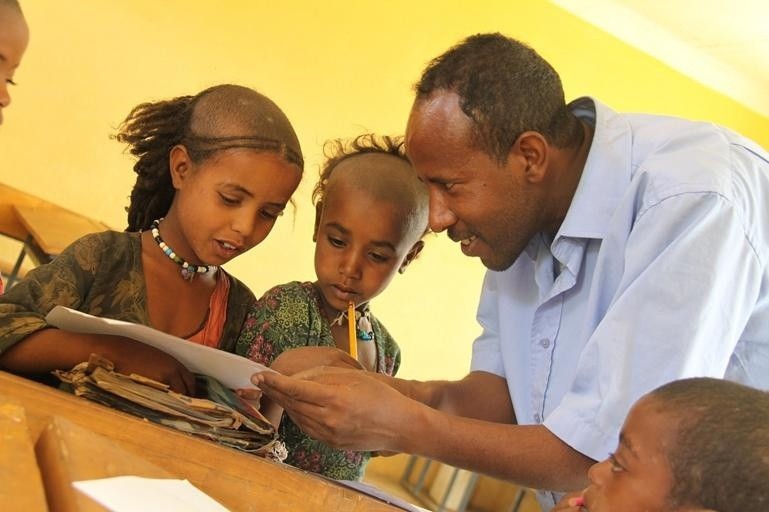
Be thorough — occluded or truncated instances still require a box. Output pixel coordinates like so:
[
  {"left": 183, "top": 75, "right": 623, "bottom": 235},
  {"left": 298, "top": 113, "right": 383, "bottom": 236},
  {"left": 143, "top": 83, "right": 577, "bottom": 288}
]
[{"left": 55, "top": 352, "right": 282, "bottom": 457}]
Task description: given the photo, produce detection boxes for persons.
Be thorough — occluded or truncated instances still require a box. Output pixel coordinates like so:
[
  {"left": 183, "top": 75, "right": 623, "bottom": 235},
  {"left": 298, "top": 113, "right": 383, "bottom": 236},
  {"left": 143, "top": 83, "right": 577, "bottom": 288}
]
[
  {"left": 1, "top": 82, "right": 310, "bottom": 409},
  {"left": 0, "top": 2, "right": 31, "bottom": 126},
  {"left": 254, "top": 32, "right": 769, "bottom": 512},
  {"left": 239, "top": 131, "right": 434, "bottom": 488},
  {"left": 552, "top": 376, "right": 769, "bottom": 511}
]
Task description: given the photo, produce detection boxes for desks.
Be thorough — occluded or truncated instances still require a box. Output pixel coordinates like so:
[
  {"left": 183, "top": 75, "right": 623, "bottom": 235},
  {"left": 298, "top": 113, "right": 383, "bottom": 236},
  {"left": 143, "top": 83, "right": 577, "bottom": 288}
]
[
  {"left": 4, "top": 204, "right": 120, "bottom": 305},
  {"left": 0, "top": 367, "right": 411, "bottom": 512}
]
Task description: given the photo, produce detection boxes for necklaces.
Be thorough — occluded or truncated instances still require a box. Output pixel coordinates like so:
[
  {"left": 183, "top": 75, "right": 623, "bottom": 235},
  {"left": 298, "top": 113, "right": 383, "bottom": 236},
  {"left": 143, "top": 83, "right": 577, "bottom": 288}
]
[{"left": 147, "top": 211, "right": 219, "bottom": 281}]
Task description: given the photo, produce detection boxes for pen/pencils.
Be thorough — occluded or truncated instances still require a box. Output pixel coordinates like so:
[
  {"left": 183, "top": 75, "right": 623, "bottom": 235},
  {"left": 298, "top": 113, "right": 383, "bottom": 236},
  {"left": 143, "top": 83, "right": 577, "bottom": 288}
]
[{"left": 348, "top": 301, "right": 358, "bottom": 361}]
